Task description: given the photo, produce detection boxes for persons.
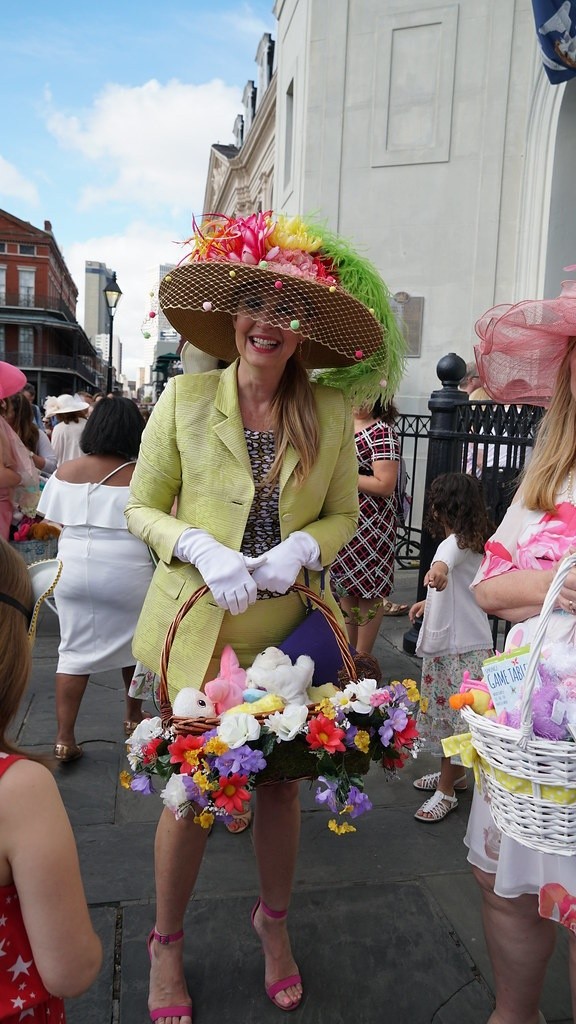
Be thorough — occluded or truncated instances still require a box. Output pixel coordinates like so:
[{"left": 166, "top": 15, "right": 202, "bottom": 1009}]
[
  {"left": 321, "top": 375, "right": 404, "bottom": 656},
  {"left": 0, "top": 539, "right": 103, "bottom": 1021},
  {"left": 408, "top": 472, "right": 496, "bottom": 823},
  {"left": 465, "top": 283, "right": 576, "bottom": 1024},
  {"left": 124, "top": 261, "right": 360, "bottom": 1024},
  {"left": 0, "top": 352, "right": 154, "bottom": 546},
  {"left": 33, "top": 389, "right": 145, "bottom": 759},
  {"left": 327, "top": 381, "right": 402, "bottom": 661}
]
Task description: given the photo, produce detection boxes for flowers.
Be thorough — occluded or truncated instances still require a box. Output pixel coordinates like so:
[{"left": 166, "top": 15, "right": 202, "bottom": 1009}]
[
  {"left": 172, "top": 210, "right": 344, "bottom": 289},
  {"left": 43, "top": 393, "right": 83, "bottom": 414},
  {"left": 120, "top": 677, "right": 431, "bottom": 836}
]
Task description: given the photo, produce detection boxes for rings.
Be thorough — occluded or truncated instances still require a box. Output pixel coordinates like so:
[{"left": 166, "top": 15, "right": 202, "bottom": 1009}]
[{"left": 569, "top": 601, "right": 573, "bottom": 609}]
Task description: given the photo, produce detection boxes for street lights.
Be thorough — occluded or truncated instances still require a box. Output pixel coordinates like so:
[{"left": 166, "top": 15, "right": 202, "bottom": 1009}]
[{"left": 102, "top": 268, "right": 123, "bottom": 394}]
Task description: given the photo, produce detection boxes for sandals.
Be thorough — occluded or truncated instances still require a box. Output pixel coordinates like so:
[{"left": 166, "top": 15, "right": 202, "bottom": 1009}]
[
  {"left": 414, "top": 790, "right": 459, "bottom": 822},
  {"left": 226, "top": 802, "right": 252, "bottom": 835},
  {"left": 382, "top": 600, "right": 409, "bottom": 615},
  {"left": 413, "top": 772, "right": 468, "bottom": 792}
]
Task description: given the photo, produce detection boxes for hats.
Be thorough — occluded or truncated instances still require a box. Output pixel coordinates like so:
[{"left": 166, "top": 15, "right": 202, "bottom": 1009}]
[
  {"left": 141, "top": 211, "right": 404, "bottom": 413},
  {"left": 475, "top": 267, "right": 576, "bottom": 408},
  {"left": 44, "top": 394, "right": 90, "bottom": 419},
  {"left": 0, "top": 361, "right": 27, "bottom": 400}
]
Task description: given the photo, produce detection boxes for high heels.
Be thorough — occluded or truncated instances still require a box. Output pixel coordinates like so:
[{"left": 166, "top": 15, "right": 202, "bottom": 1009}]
[
  {"left": 146, "top": 927, "right": 193, "bottom": 1022},
  {"left": 251, "top": 896, "right": 302, "bottom": 1011}
]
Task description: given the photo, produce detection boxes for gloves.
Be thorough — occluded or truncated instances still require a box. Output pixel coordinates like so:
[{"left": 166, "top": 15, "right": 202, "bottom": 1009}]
[
  {"left": 174, "top": 529, "right": 267, "bottom": 615},
  {"left": 250, "top": 531, "right": 321, "bottom": 594}
]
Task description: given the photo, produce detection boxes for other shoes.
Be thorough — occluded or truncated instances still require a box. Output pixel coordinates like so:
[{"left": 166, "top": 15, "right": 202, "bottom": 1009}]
[
  {"left": 124, "top": 710, "right": 152, "bottom": 734},
  {"left": 54, "top": 744, "right": 83, "bottom": 762}
]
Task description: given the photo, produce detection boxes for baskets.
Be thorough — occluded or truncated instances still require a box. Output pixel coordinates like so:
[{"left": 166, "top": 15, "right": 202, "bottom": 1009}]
[
  {"left": 156, "top": 579, "right": 365, "bottom": 789},
  {"left": 460, "top": 554, "right": 576, "bottom": 857}
]
